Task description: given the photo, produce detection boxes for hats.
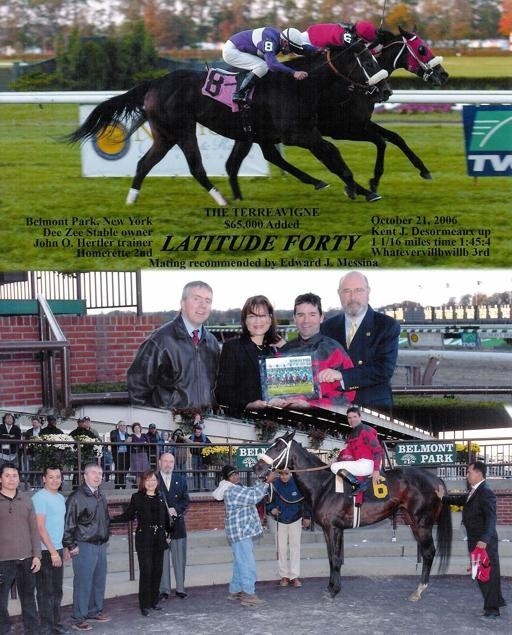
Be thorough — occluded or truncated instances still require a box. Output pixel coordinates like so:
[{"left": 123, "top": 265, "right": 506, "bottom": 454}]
[
  {"left": 222, "top": 465, "right": 239, "bottom": 480},
  {"left": 149, "top": 424, "right": 156, "bottom": 429},
  {"left": 77, "top": 416, "right": 90, "bottom": 422}
]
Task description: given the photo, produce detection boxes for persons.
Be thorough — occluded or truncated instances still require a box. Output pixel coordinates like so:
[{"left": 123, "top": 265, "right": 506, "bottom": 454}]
[
  {"left": 151, "top": 451, "right": 191, "bottom": 601},
  {"left": 1, "top": 411, "right": 214, "bottom": 493},
  {"left": 313, "top": 269, "right": 401, "bottom": 408},
  {"left": 222, "top": 26, "right": 309, "bottom": 103},
  {"left": 61, "top": 460, "right": 113, "bottom": 631},
  {"left": 433, "top": 459, "right": 507, "bottom": 620},
  {"left": 29, "top": 465, "right": 70, "bottom": 635},
  {"left": 264, "top": 466, "right": 313, "bottom": 588},
  {"left": 293, "top": 19, "right": 383, "bottom": 56},
  {"left": 0, "top": 463, "right": 43, "bottom": 635},
  {"left": 210, "top": 463, "right": 280, "bottom": 608},
  {"left": 215, "top": 293, "right": 290, "bottom": 410},
  {"left": 125, "top": 279, "right": 221, "bottom": 412},
  {"left": 271, "top": 292, "right": 356, "bottom": 409},
  {"left": 267, "top": 372, "right": 308, "bottom": 383},
  {"left": 104, "top": 467, "right": 178, "bottom": 617},
  {"left": 329, "top": 406, "right": 385, "bottom": 497}
]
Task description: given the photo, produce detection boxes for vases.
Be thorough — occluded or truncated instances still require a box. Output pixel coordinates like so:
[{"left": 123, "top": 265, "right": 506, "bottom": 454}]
[
  {"left": 51, "top": 448, "right": 66, "bottom": 464},
  {"left": 211, "top": 452, "right": 225, "bottom": 466}
]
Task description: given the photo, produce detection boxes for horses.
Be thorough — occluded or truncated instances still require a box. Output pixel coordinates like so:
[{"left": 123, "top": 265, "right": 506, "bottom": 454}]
[
  {"left": 225, "top": 24, "right": 449, "bottom": 202},
  {"left": 56, "top": 24, "right": 383, "bottom": 211},
  {"left": 251, "top": 428, "right": 452, "bottom": 603}
]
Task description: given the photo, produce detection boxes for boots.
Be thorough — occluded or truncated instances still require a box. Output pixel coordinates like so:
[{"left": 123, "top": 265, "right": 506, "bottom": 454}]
[
  {"left": 232, "top": 73, "right": 256, "bottom": 102},
  {"left": 338, "top": 469, "right": 363, "bottom": 495}
]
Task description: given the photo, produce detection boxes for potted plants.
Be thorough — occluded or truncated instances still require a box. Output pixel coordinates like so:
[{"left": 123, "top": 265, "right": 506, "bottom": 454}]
[
  {"left": 255, "top": 420, "right": 277, "bottom": 441},
  {"left": 172, "top": 408, "right": 204, "bottom": 437},
  {"left": 305, "top": 429, "right": 325, "bottom": 449}
]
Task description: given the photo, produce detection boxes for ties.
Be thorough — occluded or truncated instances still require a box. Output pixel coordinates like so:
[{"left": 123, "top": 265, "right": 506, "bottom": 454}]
[
  {"left": 347, "top": 318, "right": 356, "bottom": 346},
  {"left": 166, "top": 477, "right": 170, "bottom": 492},
  {"left": 192, "top": 330, "right": 199, "bottom": 346},
  {"left": 94, "top": 490, "right": 98, "bottom": 498}
]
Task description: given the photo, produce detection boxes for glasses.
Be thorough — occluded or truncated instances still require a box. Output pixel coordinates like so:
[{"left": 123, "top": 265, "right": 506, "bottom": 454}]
[
  {"left": 341, "top": 289, "right": 368, "bottom": 297},
  {"left": 246, "top": 313, "right": 272, "bottom": 322}
]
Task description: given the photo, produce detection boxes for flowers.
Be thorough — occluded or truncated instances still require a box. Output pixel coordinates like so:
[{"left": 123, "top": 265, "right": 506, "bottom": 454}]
[
  {"left": 465, "top": 444, "right": 480, "bottom": 453},
  {"left": 456, "top": 444, "right": 466, "bottom": 452},
  {"left": 30, "top": 434, "right": 78, "bottom": 475},
  {"left": 451, "top": 505, "right": 463, "bottom": 512},
  {"left": 201, "top": 445, "right": 237, "bottom": 458},
  {"left": 74, "top": 434, "right": 103, "bottom": 464}
]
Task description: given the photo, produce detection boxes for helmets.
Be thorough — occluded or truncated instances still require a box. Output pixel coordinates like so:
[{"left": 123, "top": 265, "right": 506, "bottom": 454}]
[
  {"left": 281, "top": 28, "right": 303, "bottom": 50},
  {"left": 356, "top": 21, "right": 376, "bottom": 43}
]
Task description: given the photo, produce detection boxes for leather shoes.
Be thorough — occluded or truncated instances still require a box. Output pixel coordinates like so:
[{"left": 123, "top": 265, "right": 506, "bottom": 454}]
[
  {"left": 479, "top": 612, "right": 500, "bottom": 621},
  {"left": 55, "top": 577, "right": 302, "bottom": 634},
  {"left": 499, "top": 600, "right": 506, "bottom": 608}
]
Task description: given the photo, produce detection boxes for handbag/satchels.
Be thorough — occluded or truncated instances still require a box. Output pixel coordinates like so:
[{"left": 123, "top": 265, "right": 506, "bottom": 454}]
[{"left": 470, "top": 547, "right": 492, "bottom": 583}]
